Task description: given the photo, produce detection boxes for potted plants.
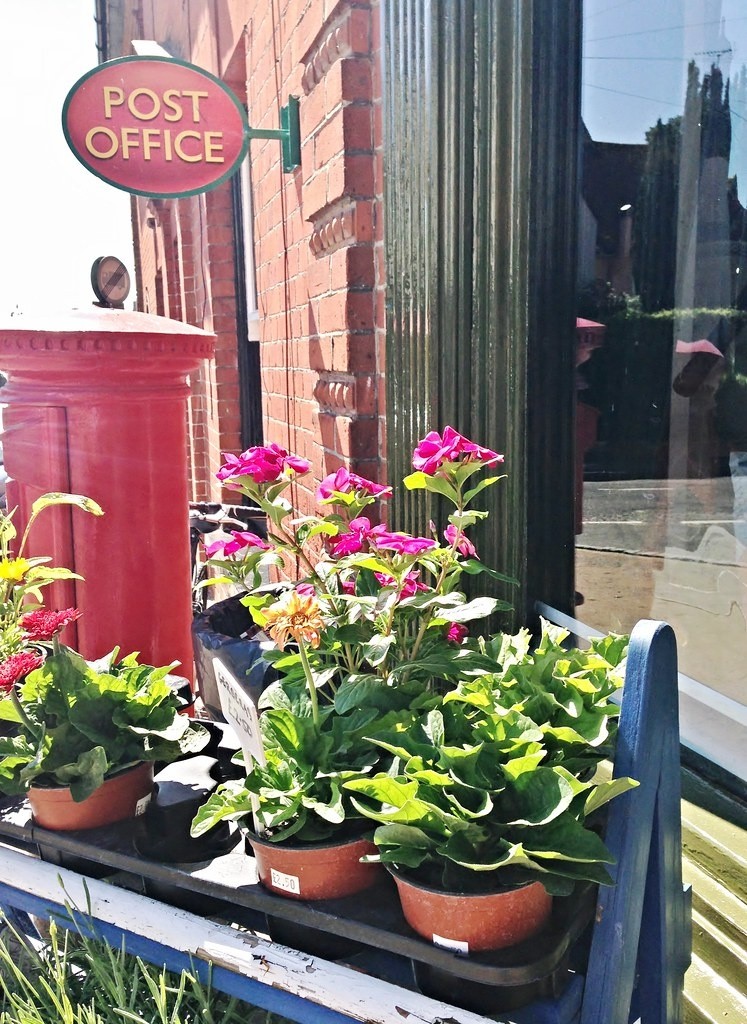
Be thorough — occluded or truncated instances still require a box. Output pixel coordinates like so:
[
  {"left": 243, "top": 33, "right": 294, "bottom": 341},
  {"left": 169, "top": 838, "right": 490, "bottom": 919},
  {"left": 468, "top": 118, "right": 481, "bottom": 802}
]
[{"left": 189, "top": 600, "right": 638, "bottom": 953}]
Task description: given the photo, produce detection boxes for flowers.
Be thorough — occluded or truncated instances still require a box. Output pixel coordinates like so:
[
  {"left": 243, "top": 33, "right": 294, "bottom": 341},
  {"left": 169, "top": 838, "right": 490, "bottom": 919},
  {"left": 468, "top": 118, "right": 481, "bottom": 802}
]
[
  {"left": 0, "top": 606, "right": 211, "bottom": 811},
  {"left": 194, "top": 428, "right": 505, "bottom": 713}
]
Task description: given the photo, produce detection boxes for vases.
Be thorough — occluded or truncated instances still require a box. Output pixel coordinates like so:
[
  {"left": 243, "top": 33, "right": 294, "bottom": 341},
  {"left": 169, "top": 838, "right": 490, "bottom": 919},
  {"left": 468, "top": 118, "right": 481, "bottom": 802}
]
[{"left": 20, "top": 755, "right": 156, "bottom": 832}]
